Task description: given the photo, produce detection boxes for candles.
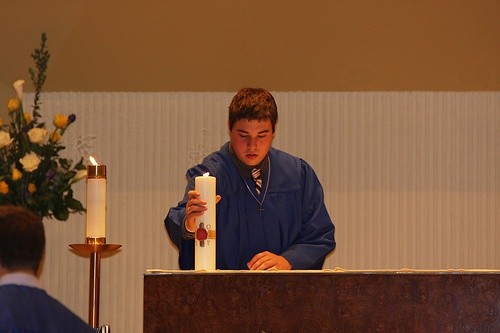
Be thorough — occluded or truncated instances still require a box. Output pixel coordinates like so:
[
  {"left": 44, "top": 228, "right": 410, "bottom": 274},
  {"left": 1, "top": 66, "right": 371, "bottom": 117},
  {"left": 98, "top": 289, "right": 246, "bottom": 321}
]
[
  {"left": 195, "top": 172, "right": 216, "bottom": 269},
  {"left": 86, "top": 155, "right": 106, "bottom": 244}
]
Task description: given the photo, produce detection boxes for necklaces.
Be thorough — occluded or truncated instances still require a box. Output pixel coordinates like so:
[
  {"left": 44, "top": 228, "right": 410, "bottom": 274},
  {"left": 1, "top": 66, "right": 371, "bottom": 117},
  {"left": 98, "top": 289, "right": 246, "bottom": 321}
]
[{"left": 228, "top": 142, "right": 271, "bottom": 217}]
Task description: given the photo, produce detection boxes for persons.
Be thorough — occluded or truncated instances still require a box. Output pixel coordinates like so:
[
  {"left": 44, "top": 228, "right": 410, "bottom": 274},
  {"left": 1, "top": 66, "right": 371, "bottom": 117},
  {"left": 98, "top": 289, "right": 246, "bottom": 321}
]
[
  {"left": 164, "top": 86, "right": 337, "bottom": 271},
  {"left": 0, "top": 205, "right": 97, "bottom": 332}
]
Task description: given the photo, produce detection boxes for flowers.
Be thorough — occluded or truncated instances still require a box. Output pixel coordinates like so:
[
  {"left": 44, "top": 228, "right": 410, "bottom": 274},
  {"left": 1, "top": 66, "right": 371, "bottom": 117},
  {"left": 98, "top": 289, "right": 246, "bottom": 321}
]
[{"left": 0, "top": 33, "right": 91, "bottom": 222}]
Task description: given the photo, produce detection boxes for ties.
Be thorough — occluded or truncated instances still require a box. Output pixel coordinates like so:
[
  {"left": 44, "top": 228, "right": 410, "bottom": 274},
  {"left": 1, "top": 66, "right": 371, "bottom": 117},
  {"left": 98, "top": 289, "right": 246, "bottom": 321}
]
[{"left": 252, "top": 168, "right": 263, "bottom": 196}]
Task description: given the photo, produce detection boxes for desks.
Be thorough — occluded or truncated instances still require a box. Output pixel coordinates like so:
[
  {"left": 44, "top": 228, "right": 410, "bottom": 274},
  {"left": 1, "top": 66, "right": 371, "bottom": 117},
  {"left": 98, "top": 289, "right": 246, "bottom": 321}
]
[{"left": 142, "top": 270, "right": 500, "bottom": 333}]
